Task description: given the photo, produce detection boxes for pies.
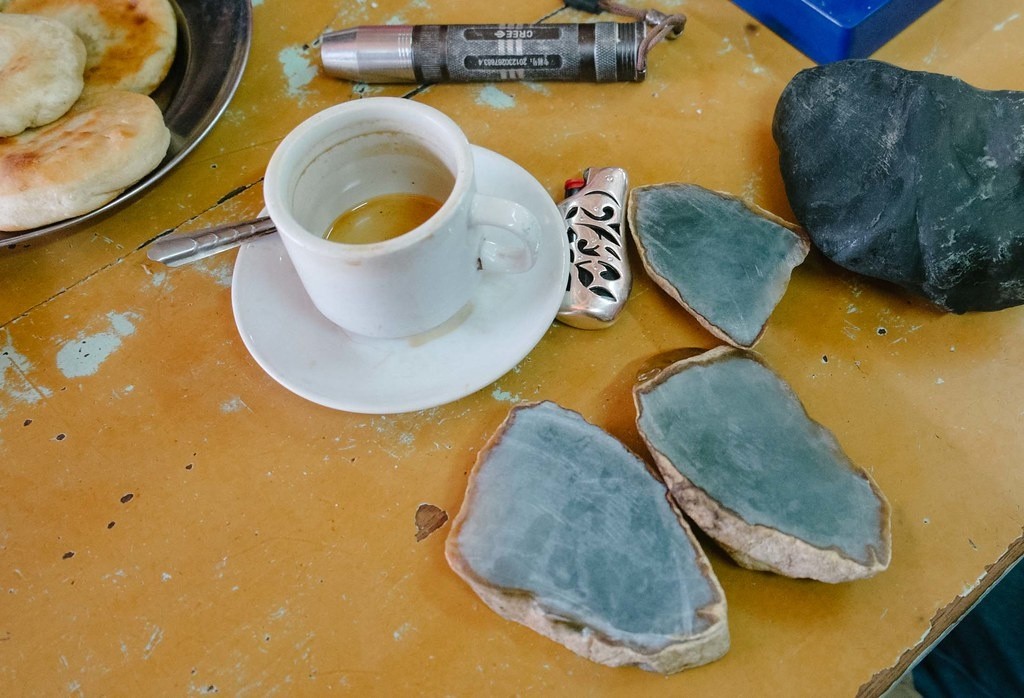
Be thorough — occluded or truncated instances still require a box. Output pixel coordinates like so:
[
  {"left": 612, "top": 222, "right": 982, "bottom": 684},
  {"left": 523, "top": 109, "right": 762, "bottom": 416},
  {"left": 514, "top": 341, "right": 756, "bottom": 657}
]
[{"left": 0, "top": 0, "right": 177, "bottom": 233}]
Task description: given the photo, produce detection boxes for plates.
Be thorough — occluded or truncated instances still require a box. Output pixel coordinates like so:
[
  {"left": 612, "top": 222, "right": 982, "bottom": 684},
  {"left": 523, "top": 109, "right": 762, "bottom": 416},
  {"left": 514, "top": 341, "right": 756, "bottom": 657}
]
[
  {"left": 0, "top": 0, "right": 254, "bottom": 257},
  {"left": 230, "top": 144, "right": 570, "bottom": 415}
]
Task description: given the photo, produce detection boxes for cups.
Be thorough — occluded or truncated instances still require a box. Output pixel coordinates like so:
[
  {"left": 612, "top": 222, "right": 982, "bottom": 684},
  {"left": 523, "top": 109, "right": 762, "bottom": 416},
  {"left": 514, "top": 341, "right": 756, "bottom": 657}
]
[{"left": 263, "top": 96, "right": 543, "bottom": 337}]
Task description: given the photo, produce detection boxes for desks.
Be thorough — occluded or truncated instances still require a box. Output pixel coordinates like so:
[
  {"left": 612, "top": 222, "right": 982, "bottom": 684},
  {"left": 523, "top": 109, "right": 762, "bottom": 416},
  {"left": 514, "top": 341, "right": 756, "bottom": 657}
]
[{"left": 0, "top": 0, "right": 1024, "bottom": 697}]
